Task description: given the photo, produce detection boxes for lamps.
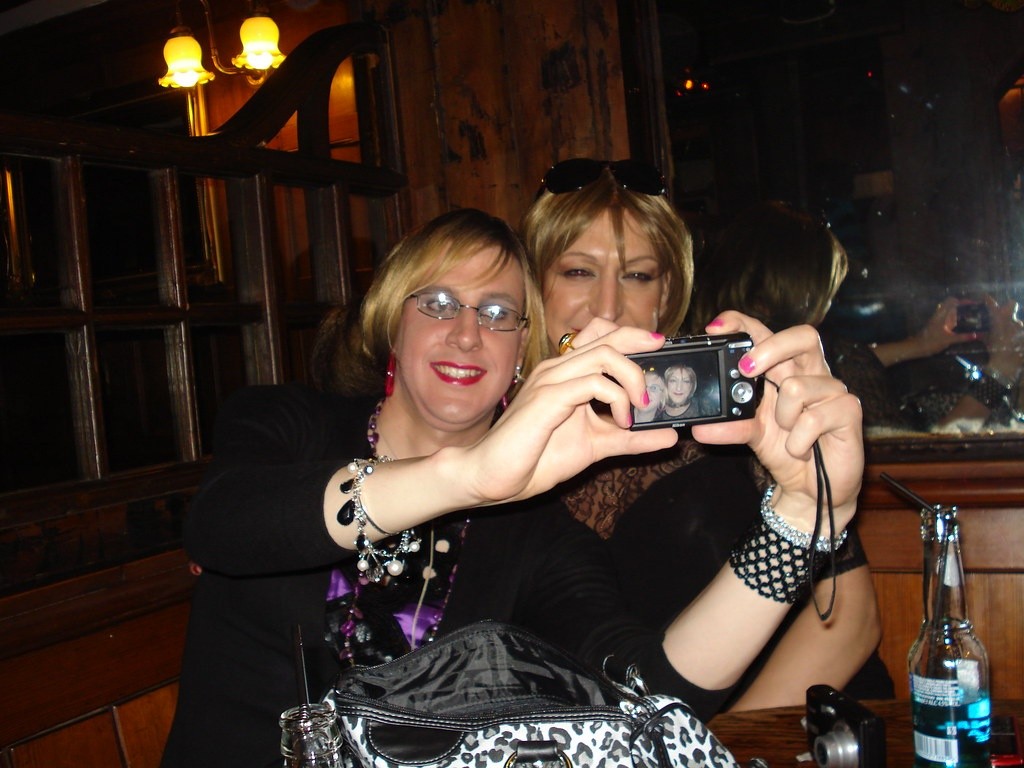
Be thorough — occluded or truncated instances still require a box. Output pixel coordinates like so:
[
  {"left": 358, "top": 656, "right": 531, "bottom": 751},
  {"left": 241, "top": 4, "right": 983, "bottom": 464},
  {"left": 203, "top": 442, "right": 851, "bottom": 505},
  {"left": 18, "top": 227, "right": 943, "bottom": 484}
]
[{"left": 157, "top": 1, "right": 288, "bottom": 89}]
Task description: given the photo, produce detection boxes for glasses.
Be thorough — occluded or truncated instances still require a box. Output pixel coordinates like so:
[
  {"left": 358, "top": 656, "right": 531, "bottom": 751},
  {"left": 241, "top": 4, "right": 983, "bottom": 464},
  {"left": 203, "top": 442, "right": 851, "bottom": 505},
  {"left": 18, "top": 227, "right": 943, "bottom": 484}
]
[
  {"left": 533, "top": 156, "right": 670, "bottom": 203},
  {"left": 644, "top": 383, "right": 661, "bottom": 392},
  {"left": 663, "top": 360, "right": 694, "bottom": 371},
  {"left": 776, "top": 200, "right": 833, "bottom": 241},
  {"left": 407, "top": 291, "right": 529, "bottom": 332}
]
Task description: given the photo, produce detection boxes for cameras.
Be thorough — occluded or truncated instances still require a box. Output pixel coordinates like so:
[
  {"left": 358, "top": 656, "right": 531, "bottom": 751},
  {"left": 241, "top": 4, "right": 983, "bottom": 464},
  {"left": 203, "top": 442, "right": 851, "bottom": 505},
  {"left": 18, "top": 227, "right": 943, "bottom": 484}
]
[
  {"left": 806, "top": 684, "right": 886, "bottom": 768},
  {"left": 949, "top": 304, "right": 992, "bottom": 333},
  {"left": 610, "top": 331, "right": 763, "bottom": 431}
]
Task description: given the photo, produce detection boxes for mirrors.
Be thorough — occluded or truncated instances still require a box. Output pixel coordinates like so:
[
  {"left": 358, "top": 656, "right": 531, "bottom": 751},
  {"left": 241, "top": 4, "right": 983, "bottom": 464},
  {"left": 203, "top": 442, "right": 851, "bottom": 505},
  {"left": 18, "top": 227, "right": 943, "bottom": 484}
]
[{"left": 614, "top": 1, "right": 1023, "bottom": 468}]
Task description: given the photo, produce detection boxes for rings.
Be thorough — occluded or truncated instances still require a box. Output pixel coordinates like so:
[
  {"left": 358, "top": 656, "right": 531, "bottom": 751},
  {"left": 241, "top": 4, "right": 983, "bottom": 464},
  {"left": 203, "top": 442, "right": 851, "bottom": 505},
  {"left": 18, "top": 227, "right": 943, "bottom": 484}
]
[
  {"left": 558, "top": 331, "right": 578, "bottom": 356},
  {"left": 936, "top": 302, "right": 943, "bottom": 311}
]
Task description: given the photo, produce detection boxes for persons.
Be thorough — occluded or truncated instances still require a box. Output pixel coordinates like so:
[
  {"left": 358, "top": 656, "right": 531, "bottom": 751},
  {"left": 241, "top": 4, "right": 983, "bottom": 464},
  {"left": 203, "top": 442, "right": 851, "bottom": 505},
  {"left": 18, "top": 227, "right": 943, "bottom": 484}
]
[
  {"left": 633, "top": 370, "right": 668, "bottom": 424},
  {"left": 682, "top": 202, "right": 920, "bottom": 439},
  {"left": 518, "top": 160, "right": 883, "bottom": 715},
  {"left": 872, "top": 206, "right": 1023, "bottom": 436},
  {"left": 659, "top": 364, "right": 703, "bottom": 421},
  {"left": 160, "top": 210, "right": 862, "bottom": 768}
]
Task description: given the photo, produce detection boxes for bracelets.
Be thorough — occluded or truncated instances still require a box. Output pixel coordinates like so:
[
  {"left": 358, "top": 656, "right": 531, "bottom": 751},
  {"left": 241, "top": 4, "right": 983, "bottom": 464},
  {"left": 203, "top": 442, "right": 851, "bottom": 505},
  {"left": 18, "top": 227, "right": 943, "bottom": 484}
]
[
  {"left": 965, "top": 365, "right": 1013, "bottom": 410},
  {"left": 331, "top": 455, "right": 420, "bottom": 585},
  {"left": 728, "top": 483, "right": 849, "bottom": 605}
]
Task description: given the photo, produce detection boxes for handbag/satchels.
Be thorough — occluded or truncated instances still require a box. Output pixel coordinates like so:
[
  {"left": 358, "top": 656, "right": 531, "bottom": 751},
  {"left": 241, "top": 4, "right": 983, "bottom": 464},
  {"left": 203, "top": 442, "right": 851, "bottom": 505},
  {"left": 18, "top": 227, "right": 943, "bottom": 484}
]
[{"left": 323, "top": 619, "right": 744, "bottom": 767}]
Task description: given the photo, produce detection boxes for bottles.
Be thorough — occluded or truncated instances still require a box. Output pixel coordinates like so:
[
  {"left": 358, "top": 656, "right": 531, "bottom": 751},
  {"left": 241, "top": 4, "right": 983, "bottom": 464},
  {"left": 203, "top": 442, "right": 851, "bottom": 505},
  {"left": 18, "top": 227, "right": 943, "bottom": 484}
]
[
  {"left": 911, "top": 503, "right": 993, "bottom": 768},
  {"left": 279, "top": 704, "right": 361, "bottom": 767}
]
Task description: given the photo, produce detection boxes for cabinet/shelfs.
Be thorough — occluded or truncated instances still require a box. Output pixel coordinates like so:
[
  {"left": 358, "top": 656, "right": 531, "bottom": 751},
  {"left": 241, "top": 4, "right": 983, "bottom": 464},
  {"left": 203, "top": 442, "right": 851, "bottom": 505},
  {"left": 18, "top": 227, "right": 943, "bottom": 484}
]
[{"left": 857, "top": 460, "right": 1024, "bottom": 702}]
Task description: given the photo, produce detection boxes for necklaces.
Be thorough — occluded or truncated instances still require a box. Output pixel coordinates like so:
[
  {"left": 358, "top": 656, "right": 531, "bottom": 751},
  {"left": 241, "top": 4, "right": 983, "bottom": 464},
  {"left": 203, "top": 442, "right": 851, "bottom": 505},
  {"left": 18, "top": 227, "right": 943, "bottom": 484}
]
[{"left": 334, "top": 395, "right": 475, "bottom": 670}]
